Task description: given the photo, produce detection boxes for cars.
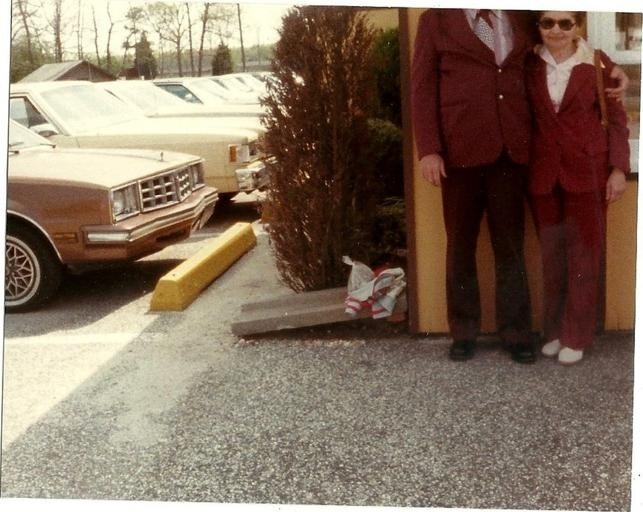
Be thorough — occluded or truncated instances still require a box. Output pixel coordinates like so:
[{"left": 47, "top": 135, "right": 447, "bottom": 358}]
[
  {"left": 7, "top": 115, "right": 226, "bottom": 318},
  {"left": 10, "top": 80, "right": 280, "bottom": 211},
  {"left": 110, "top": 69, "right": 302, "bottom": 121}
]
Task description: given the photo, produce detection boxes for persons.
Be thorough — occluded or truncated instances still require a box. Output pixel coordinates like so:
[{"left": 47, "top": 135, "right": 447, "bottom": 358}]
[
  {"left": 408, "top": 8, "right": 633, "bottom": 365},
  {"left": 526, "top": 11, "right": 632, "bottom": 367}
]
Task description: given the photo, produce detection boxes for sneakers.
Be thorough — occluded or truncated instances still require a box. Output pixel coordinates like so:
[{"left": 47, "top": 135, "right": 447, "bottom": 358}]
[
  {"left": 541, "top": 338, "right": 561, "bottom": 357},
  {"left": 556, "top": 346, "right": 586, "bottom": 364}
]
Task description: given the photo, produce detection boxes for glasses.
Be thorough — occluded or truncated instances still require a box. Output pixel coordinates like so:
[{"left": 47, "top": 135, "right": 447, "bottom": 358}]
[{"left": 539, "top": 17, "right": 577, "bottom": 30}]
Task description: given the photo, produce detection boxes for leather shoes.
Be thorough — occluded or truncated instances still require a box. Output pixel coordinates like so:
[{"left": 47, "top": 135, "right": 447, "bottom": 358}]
[
  {"left": 448, "top": 340, "right": 475, "bottom": 360},
  {"left": 503, "top": 338, "right": 535, "bottom": 363}
]
